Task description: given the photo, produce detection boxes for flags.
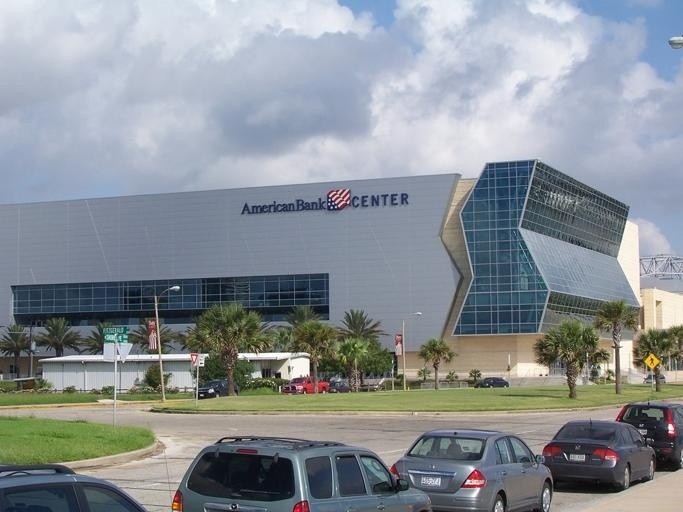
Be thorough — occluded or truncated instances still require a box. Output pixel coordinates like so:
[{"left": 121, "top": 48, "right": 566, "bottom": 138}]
[{"left": 326, "top": 188, "right": 350, "bottom": 212}]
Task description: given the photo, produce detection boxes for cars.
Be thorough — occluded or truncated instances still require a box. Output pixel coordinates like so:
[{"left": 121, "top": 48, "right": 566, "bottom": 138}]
[
  {"left": 390, "top": 429, "right": 555, "bottom": 511},
  {"left": 173, "top": 435, "right": 431, "bottom": 510},
  {"left": 0, "top": 464, "right": 145, "bottom": 511},
  {"left": 195, "top": 378, "right": 238, "bottom": 398},
  {"left": 616, "top": 402, "right": 682, "bottom": 468},
  {"left": 282, "top": 377, "right": 351, "bottom": 393},
  {"left": 544, "top": 421, "right": 655, "bottom": 489},
  {"left": 474, "top": 377, "right": 509, "bottom": 388}
]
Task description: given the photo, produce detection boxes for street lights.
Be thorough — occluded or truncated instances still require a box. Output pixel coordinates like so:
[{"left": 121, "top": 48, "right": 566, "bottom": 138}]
[
  {"left": 154, "top": 286, "right": 180, "bottom": 398},
  {"left": 402, "top": 312, "right": 422, "bottom": 389}
]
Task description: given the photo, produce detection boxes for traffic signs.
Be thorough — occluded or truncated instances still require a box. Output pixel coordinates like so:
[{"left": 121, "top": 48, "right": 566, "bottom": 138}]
[{"left": 103, "top": 326, "right": 129, "bottom": 343}]
